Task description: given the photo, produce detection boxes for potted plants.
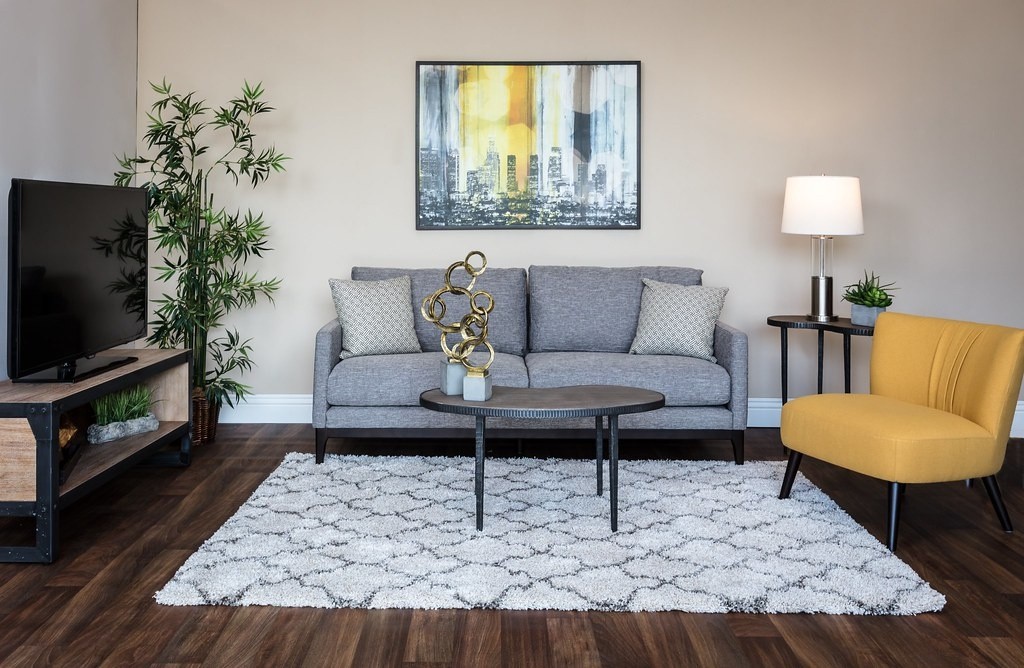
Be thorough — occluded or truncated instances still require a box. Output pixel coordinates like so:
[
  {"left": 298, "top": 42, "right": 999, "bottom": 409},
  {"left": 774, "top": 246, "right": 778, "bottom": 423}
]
[
  {"left": 87, "top": 381, "right": 170, "bottom": 446},
  {"left": 839, "top": 270, "right": 901, "bottom": 328},
  {"left": 113, "top": 77, "right": 294, "bottom": 446}
]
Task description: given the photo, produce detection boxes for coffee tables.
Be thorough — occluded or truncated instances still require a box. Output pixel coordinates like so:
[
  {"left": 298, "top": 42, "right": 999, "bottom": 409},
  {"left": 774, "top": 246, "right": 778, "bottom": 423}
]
[{"left": 417, "top": 385, "right": 666, "bottom": 532}]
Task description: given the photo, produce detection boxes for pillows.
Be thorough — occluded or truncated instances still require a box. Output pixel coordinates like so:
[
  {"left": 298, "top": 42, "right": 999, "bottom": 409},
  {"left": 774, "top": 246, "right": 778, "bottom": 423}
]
[
  {"left": 628, "top": 278, "right": 730, "bottom": 364},
  {"left": 326, "top": 274, "right": 423, "bottom": 359}
]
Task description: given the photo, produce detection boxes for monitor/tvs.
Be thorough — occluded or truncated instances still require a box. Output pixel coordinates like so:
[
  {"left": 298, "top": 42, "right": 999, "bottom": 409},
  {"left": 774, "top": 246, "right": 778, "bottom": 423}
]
[{"left": 8, "top": 177, "right": 148, "bottom": 382}]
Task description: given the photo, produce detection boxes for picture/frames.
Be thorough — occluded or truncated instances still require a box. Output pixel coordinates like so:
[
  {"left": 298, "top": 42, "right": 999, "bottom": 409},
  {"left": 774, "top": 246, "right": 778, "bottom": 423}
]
[{"left": 414, "top": 60, "right": 641, "bottom": 229}]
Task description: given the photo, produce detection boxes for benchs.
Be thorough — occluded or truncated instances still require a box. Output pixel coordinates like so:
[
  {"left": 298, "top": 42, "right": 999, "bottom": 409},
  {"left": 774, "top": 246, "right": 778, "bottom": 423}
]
[{"left": 313, "top": 265, "right": 749, "bottom": 466}]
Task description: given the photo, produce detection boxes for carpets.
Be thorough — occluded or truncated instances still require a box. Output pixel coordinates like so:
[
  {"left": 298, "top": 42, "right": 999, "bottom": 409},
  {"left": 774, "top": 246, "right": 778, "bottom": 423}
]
[{"left": 152, "top": 451, "right": 947, "bottom": 618}]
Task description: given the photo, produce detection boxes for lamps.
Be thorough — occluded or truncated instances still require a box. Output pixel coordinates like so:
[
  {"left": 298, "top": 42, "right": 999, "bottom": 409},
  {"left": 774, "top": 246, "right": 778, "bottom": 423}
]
[{"left": 779, "top": 174, "right": 864, "bottom": 323}]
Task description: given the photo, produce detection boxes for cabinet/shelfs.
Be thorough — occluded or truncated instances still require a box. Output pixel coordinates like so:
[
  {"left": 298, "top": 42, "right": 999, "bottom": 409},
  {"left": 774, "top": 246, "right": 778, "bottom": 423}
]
[{"left": 0, "top": 350, "right": 194, "bottom": 565}]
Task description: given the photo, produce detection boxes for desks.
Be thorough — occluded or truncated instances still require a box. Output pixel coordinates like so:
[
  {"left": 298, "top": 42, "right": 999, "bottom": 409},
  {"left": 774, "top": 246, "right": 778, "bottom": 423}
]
[{"left": 767, "top": 315, "right": 875, "bottom": 454}]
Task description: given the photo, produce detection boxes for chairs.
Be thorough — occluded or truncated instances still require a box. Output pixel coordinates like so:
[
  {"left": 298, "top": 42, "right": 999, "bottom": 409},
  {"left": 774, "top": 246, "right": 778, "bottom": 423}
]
[{"left": 779, "top": 311, "right": 1024, "bottom": 554}]
